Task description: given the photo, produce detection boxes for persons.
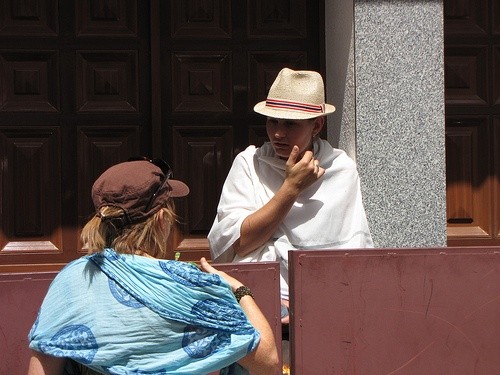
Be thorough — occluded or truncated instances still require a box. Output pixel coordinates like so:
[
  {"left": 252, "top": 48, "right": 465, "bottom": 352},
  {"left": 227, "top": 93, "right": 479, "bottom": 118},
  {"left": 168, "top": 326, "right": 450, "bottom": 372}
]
[
  {"left": 28, "top": 155, "right": 279, "bottom": 375},
  {"left": 206, "top": 68, "right": 374, "bottom": 363}
]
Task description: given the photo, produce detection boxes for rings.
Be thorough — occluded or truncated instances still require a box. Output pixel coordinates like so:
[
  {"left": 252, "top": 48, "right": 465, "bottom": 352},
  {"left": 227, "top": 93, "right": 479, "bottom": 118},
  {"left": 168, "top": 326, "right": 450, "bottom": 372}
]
[{"left": 313, "top": 163, "right": 319, "bottom": 169}]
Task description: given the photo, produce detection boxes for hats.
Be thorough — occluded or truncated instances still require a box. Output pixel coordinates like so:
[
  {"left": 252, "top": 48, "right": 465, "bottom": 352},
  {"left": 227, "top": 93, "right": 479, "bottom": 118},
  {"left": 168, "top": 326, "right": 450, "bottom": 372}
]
[
  {"left": 251, "top": 67, "right": 337, "bottom": 120},
  {"left": 90, "top": 161, "right": 189, "bottom": 227}
]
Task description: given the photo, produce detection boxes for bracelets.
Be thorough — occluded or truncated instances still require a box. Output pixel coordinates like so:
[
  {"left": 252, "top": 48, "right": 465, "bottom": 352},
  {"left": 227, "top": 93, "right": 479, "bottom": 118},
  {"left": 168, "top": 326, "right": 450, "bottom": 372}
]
[{"left": 235, "top": 284, "right": 255, "bottom": 302}]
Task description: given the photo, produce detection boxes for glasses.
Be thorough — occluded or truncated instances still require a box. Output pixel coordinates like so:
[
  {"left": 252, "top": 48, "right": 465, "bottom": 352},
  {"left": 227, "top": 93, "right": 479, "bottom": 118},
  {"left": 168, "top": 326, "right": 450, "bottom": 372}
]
[{"left": 145, "top": 162, "right": 173, "bottom": 212}]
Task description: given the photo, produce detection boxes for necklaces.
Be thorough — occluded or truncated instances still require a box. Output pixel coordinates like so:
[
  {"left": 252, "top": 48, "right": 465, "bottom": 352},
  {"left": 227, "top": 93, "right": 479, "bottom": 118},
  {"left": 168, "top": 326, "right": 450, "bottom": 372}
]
[{"left": 134, "top": 245, "right": 149, "bottom": 253}]
